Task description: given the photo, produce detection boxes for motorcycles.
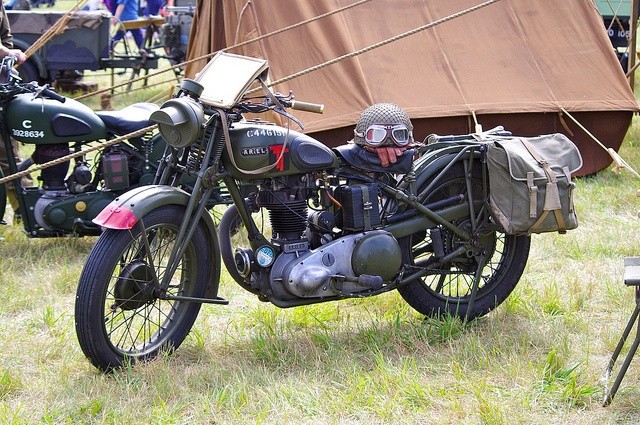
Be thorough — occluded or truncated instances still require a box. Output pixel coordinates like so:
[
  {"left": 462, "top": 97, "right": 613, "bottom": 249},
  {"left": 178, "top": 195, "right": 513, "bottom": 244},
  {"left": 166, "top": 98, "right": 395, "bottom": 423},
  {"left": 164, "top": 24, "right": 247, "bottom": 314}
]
[
  {"left": 73, "top": 77, "right": 531, "bottom": 374},
  {"left": 0, "top": 55, "right": 258, "bottom": 239}
]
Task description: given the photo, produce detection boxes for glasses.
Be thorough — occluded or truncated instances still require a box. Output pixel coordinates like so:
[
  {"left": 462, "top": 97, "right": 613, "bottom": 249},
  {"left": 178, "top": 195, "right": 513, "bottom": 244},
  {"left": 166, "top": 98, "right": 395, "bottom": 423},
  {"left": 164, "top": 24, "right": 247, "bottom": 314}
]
[{"left": 364, "top": 124, "right": 411, "bottom": 149}]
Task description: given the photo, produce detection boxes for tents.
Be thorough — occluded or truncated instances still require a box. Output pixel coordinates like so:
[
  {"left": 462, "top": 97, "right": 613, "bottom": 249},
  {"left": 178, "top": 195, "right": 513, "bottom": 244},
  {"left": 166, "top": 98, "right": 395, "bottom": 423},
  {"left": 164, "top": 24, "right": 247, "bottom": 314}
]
[{"left": 181, "top": 0, "right": 640, "bottom": 180}]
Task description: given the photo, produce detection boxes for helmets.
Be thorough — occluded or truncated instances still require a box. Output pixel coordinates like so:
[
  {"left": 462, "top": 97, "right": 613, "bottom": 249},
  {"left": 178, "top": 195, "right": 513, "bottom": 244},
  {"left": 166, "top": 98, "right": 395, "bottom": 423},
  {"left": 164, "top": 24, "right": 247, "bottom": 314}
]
[{"left": 352, "top": 103, "right": 413, "bottom": 149}]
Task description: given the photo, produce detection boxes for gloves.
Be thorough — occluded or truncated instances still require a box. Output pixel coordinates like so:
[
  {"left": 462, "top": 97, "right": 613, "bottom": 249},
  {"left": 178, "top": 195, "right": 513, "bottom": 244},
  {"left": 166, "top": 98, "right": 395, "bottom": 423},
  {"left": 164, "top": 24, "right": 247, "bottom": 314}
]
[{"left": 365, "top": 146, "right": 406, "bottom": 167}]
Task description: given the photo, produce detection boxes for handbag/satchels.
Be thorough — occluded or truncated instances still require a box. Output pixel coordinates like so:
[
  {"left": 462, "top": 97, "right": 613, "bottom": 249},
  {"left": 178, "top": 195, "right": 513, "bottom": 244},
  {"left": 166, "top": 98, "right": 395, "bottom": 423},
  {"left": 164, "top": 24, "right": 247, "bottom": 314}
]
[{"left": 485, "top": 132, "right": 583, "bottom": 236}]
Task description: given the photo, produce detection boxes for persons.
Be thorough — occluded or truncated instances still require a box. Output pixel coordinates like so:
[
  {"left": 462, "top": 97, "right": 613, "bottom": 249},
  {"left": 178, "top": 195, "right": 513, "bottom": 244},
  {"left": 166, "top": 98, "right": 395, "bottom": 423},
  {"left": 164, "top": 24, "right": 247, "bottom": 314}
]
[
  {"left": 145, "top": 0, "right": 175, "bottom": 17},
  {"left": 0, "top": 1, "right": 38, "bottom": 227},
  {"left": 110, "top": 0, "right": 144, "bottom": 50}
]
